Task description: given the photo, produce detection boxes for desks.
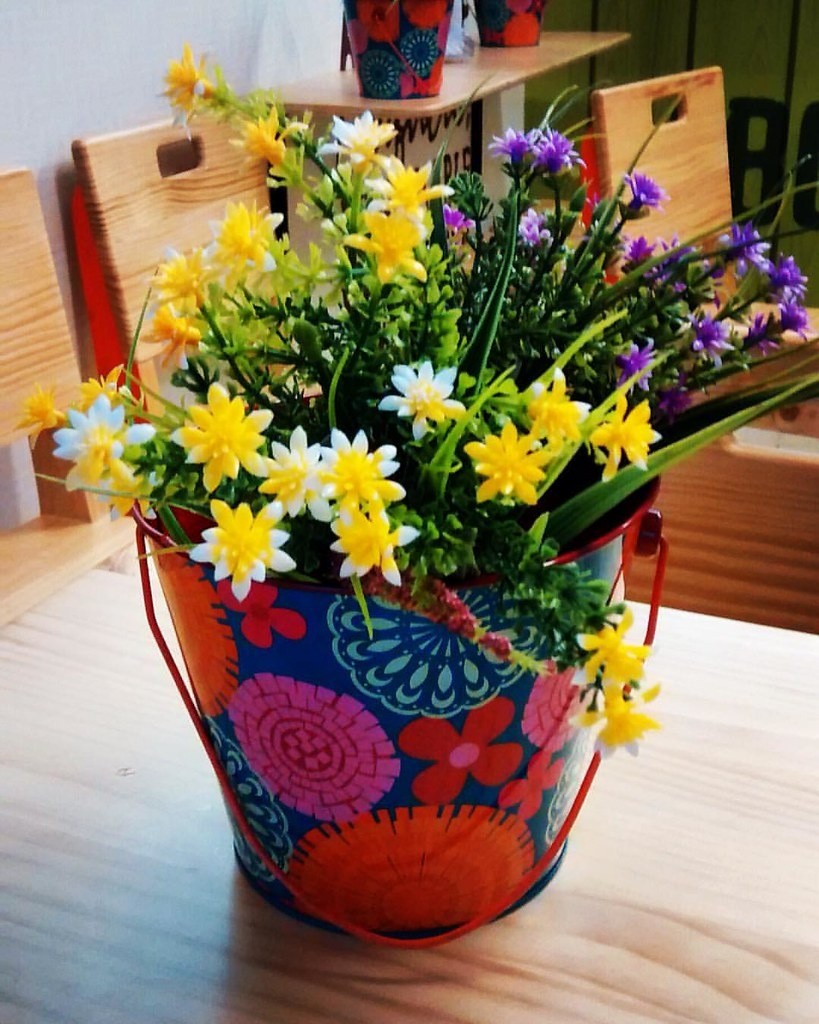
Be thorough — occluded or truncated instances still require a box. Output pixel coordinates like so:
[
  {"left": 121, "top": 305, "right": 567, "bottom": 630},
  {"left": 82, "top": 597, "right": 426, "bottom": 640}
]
[{"left": 265, "top": 32, "right": 631, "bottom": 260}]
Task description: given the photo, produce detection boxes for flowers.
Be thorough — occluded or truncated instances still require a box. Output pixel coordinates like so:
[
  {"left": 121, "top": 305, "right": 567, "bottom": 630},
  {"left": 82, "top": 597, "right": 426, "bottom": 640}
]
[{"left": 10, "top": 44, "right": 819, "bottom": 756}]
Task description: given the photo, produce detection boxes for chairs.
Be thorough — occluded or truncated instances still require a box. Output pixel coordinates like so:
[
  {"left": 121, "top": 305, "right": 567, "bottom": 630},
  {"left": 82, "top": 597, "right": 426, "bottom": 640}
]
[
  {"left": 1, "top": 537, "right": 819, "bottom": 1024},
  {"left": 0, "top": 114, "right": 271, "bottom": 636},
  {"left": 590, "top": 66, "right": 818, "bottom": 634}
]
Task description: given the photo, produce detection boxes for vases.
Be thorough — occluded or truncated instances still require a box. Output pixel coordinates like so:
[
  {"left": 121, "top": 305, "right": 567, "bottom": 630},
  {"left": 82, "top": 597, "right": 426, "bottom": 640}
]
[{"left": 130, "top": 474, "right": 669, "bottom": 951}]
